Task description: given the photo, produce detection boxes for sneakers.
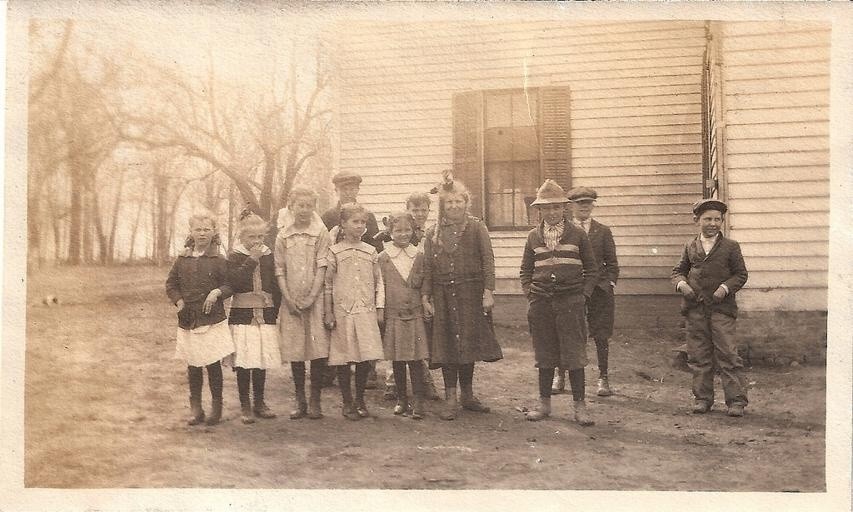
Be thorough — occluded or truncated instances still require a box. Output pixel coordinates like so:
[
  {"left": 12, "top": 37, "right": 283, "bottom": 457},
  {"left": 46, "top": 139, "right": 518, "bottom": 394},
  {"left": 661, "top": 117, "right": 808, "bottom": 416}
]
[
  {"left": 317, "top": 374, "right": 380, "bottom": 393},
  {"left": 724, "top": 401, "right": 745, "bottom": 417},
  {"left": 596, "top": 378, "right": 612, "bottom": 396},
  {"left": 692, "top": 401, "right": 712, "bottom": 415},
  {"left": 549, "top": 378, "right": 566, "bottom": 394},
  {"left": 343, "top": 397, "right": 369, "bottom": 420}
]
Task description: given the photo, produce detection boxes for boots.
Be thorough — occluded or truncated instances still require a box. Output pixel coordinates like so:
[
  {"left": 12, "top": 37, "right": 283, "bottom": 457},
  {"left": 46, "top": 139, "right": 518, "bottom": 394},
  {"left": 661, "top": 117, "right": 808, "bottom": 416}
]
[
  {"left": 573, "top": 398, "right": 595, "bottom": 427},
  {"left": 289, "top": 384, "right": 321, "bottom": 421},
  {"left": 438, "top": 385, "right": 459, "bottom": 420},
  {"left": 240, "top": 387, "right": 276, "bottom": 425},
  {"left": 527, "top": 395, "right": 552, "bottom": 421},
  {"left": 391, "top": 387, "right": 427, "bottom": 420},
  {"left": 186, "top": 390, "right": 222, "bottom": 424},
  {"left": 459, "top": 379, "right": 490, "bottom": 416}
]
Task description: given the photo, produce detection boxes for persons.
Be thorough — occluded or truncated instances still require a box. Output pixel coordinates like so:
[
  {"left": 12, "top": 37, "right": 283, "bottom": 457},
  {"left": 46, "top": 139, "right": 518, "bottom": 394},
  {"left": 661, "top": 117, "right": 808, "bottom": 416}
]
[
  {"left": 670, "top": 199, "right": 749, "bottom": 416},
  {"left": 550, "top": 186, "right": 620, "bottom": 395},
  {"left": 166, "top": 209, "right": 236, "bottom": 425},
  {"left": 519, "top": 178, "right": 597, "bottom": 427},
  {"left": 226, "top": 170, "right": 503, "bottom": 419}
]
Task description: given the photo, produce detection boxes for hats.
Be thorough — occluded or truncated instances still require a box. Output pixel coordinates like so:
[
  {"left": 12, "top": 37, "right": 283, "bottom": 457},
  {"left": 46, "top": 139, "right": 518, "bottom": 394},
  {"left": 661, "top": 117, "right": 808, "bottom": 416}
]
[
  {"left": 567, "top": 187, "right": 598, "bottom": 205},
  {"left": 691, "top": 199, "right": 729, "bottom": 218},
  {"left": 331, "top": 171, "right": 362, "bottom": 186},
  {"left": 530, "top": 178, "right": 572, "bottom": 210}
]
